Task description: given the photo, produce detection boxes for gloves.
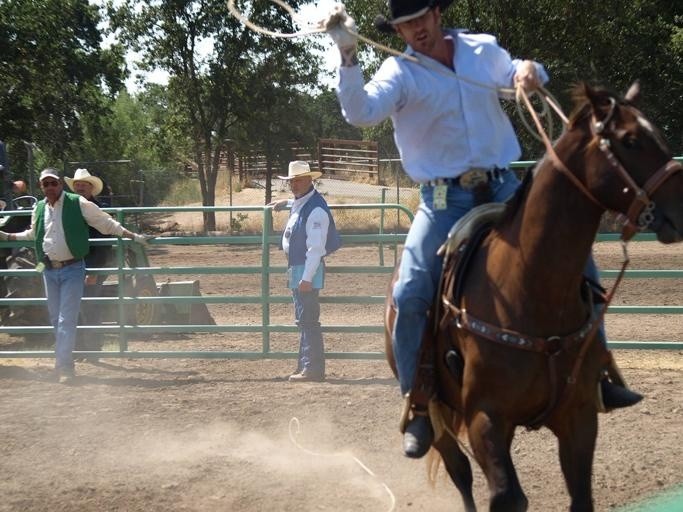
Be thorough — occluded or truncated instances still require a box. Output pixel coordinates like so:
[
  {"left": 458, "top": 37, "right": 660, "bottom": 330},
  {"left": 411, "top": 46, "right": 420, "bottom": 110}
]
[
  {"left": 134, "top": 232, "right": 155, "bottom": 246},
  {"left": 0, "top": 230, "right": 10, "bottom": 241}
]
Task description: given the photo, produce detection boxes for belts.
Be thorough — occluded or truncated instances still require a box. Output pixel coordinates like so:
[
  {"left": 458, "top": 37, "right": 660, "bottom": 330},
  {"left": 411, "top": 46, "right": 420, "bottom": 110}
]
[
  {"left": 50, "top": 258, "right": 82, "bottom": 270},
  {"left": 419, "top": 166, "right": 510, "bottom": 191}
]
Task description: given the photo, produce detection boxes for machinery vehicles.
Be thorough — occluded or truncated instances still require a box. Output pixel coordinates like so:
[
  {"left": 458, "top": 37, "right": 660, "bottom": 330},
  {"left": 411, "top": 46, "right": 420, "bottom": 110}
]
[{"left": 0, "top": 195, "right": 219, "bottom": 336}]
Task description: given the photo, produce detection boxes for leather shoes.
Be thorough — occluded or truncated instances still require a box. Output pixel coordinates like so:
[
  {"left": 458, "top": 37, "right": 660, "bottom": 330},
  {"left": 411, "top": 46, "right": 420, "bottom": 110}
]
[
  {"left": 403, "top": 403, "right": 433, "bottom": 459},
  {"left": 601, "top": 377, "right": 642, "bottom": 407}
]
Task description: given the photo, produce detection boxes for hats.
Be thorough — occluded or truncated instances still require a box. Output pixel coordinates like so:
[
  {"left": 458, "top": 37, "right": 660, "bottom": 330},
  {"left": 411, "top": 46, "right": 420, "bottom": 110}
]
[
  {"left": 39, "top": 167, "right": 62, "bottom": 181},
  {"left": 276, "top": 161, "right": 323, "bottom": 180},
  {"left": 63, "top": 169, "right": 104, "bottom": 197},
  {"left": 374, "top": 1, "right": 452, "bottom": 33}
]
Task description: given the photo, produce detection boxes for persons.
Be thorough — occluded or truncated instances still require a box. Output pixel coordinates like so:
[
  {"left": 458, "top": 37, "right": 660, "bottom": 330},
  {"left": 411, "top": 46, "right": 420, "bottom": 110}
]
[
  {"left": 0, "top": 141, "right": 156, "bottom": 383},
  {"left": 325, "top": 0, "right": 642, "bottom": 457},
  {"left": 267, "top": 160, "right": 342, "bottom": 383}
]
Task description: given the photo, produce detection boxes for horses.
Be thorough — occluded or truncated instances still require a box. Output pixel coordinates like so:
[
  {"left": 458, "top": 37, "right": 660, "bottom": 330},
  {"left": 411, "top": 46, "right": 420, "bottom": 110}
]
[{"left": 381, "top": 76, "right": 682, "bottom": 512}]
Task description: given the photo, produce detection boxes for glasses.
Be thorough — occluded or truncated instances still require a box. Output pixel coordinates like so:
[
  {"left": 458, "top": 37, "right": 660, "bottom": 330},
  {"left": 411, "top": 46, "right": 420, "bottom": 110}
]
[{"left": 43, "top": 180, "right": 59, "bottom": 187}]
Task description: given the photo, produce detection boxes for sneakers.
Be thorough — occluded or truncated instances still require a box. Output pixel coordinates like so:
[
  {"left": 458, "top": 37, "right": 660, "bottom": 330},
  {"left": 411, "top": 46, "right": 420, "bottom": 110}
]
[
  {"left": 56, "top": 368, "right": 72, "bottom": 384},
  {"left": 282, "top": 369, "right": 324, "bottom": 383}
]
